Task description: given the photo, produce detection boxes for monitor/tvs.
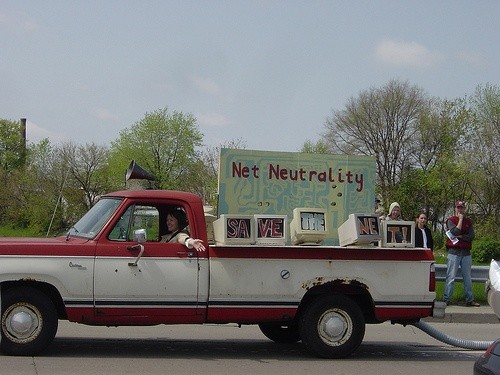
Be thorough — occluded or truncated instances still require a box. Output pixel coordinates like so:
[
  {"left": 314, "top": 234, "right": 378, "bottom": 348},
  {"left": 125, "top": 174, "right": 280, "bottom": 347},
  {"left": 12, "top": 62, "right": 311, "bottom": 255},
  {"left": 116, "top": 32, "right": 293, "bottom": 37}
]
[
  {"left": 338, "top": 213, "right": 383, "bottom": 248},
  {"left": 378, "top": 221, "right": 415, "bottom": 248},
  {"left": 213, "top": 214, "right": 287, "bottom": 247},
  {"left": 290, "top": 208, "right": 328, "bottom": 246}
]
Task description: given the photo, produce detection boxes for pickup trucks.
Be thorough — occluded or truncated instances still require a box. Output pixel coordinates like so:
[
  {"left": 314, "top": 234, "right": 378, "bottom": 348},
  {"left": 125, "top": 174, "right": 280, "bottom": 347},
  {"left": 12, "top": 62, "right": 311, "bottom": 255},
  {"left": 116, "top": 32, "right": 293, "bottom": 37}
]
[{"left": 1, "top": 190, "right": 436, "bottom": 358}]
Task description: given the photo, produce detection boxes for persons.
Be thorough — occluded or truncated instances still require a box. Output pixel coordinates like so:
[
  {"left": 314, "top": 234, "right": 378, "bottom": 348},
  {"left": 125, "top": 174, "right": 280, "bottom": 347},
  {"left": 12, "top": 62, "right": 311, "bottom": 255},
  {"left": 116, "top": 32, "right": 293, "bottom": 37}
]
[
  {"left": 160, "top": 210, "right": 206, "bottom": 252},
  {"left": 118, "top": 226, "right": 126, "bottom": 239},
  {"left": 386, "top": 202, "right": 434, "bottom": 253},
  {"left": 443, "top": 201, "right": 481, "bottom": 307}
]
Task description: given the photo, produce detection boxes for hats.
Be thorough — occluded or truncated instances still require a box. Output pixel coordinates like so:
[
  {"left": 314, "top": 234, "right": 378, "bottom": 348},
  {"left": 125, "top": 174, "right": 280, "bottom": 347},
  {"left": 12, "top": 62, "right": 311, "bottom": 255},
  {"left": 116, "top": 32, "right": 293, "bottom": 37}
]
[{"left": 455, "top": 200, "right": 466, "bottom": 208}]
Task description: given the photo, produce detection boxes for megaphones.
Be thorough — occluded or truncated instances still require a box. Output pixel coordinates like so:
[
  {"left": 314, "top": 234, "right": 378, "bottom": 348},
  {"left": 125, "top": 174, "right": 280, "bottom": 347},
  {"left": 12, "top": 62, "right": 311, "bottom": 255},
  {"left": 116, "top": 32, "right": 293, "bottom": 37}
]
[{"left": 126, "top": 160, "right": 156, "bottom": 181}]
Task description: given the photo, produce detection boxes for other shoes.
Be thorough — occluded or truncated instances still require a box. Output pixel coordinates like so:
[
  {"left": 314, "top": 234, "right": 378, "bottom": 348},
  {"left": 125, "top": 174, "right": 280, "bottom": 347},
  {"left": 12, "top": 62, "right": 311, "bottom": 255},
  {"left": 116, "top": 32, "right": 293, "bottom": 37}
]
[
  {"left": 444, "top": 300, "right": 452, "bottom": 305},
  {"left": 467, "top": 301, "right": 480, "bottom": 307}
]
